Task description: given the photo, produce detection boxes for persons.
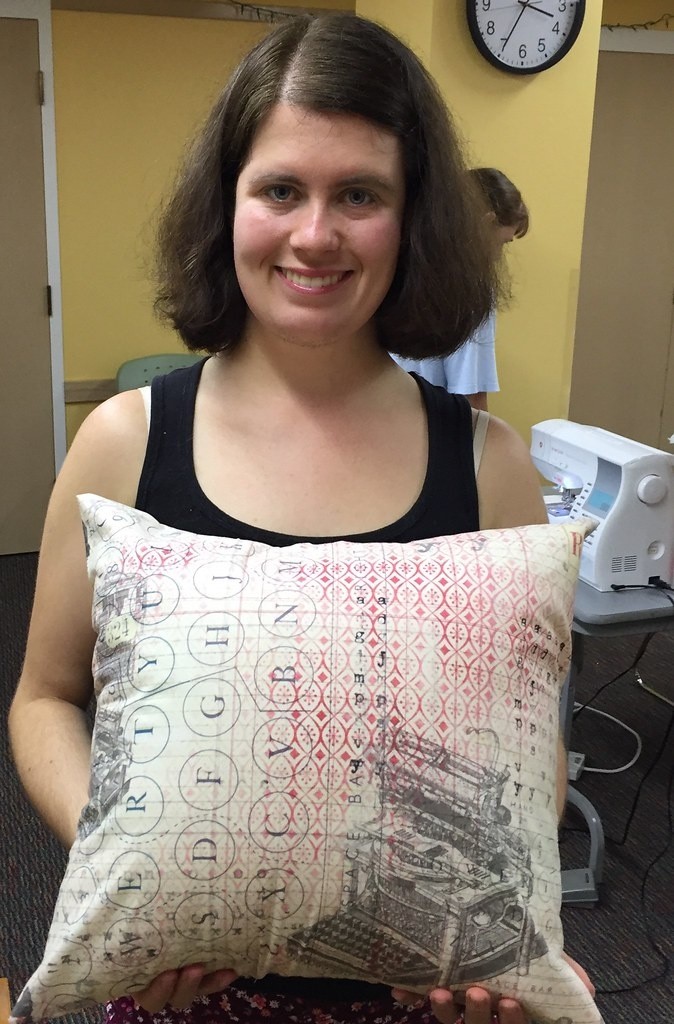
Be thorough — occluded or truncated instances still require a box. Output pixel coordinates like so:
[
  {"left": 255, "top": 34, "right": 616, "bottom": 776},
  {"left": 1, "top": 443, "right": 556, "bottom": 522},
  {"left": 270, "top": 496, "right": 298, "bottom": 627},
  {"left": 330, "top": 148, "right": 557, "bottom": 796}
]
[
  {"left": 4, "top": 8, "right": 598, "bottom": 1022},
  {"left": 381, "top": 166, "right": 533, "bottom": 413}
]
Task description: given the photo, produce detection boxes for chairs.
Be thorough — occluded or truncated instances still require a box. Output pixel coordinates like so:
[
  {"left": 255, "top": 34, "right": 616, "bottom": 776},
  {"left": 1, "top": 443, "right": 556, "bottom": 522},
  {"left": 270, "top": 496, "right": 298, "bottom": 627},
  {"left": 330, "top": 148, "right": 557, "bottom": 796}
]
[{"left": 114, "top": 352, "right": 210, "bottom": 395}]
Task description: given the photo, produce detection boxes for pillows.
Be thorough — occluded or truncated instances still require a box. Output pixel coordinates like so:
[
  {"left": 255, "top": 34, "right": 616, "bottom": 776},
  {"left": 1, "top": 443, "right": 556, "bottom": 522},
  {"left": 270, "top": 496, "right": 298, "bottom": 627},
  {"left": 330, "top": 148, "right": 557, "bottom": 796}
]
[{"left": 7, "top": 493, "right": 610, "bottom": 1024}]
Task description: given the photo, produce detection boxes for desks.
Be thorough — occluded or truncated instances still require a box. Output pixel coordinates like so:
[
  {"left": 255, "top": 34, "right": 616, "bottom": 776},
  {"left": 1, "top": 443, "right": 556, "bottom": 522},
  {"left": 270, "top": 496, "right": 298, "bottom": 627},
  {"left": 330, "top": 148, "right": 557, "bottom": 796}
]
[{"left": 531, "top": 485, "right": 672, "bottom": 905}]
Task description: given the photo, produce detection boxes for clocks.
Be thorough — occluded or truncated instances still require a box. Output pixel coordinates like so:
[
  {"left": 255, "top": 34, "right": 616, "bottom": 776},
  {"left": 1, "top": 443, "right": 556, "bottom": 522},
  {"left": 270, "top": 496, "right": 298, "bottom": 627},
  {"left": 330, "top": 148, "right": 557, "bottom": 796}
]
[{"left": 466, "top": 1, "right": 586, "bottom": 75}]
